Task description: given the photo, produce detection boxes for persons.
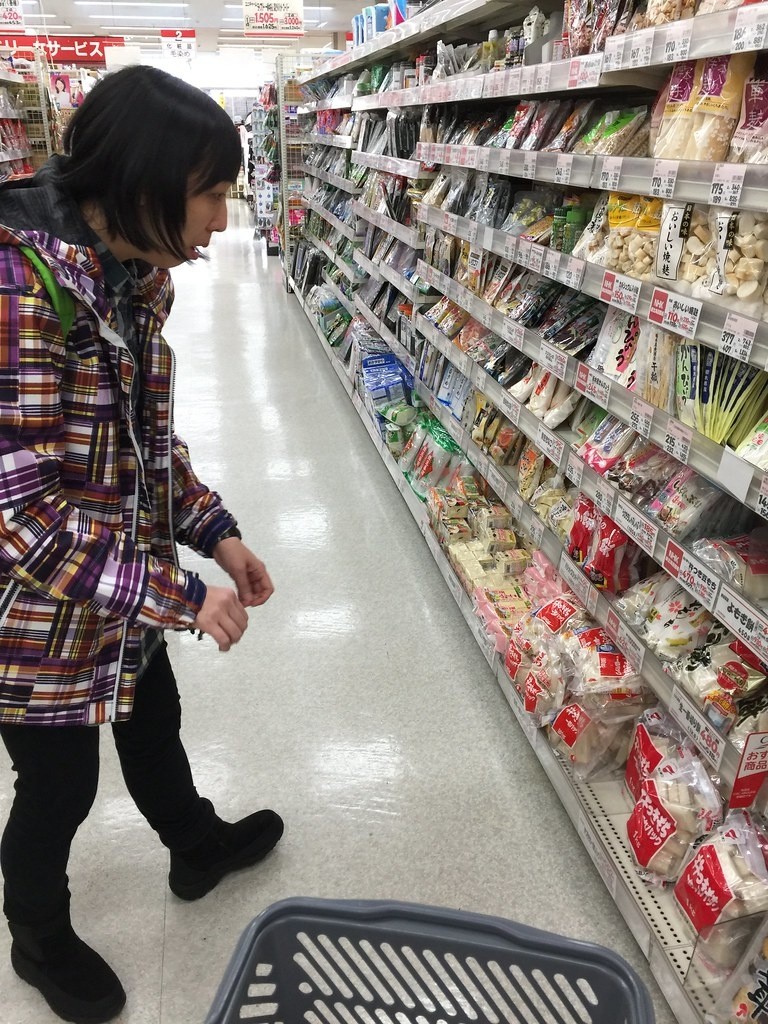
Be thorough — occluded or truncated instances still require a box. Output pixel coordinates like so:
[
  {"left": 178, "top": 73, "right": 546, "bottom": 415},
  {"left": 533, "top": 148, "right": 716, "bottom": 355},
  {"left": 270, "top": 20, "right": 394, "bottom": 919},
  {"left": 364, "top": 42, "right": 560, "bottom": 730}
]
[
  {"left": 1, "top": 63, "right": 284, "bottom": 1023},
  {"left": 54, "top": 77, "right": 70, "bottom": 107}
]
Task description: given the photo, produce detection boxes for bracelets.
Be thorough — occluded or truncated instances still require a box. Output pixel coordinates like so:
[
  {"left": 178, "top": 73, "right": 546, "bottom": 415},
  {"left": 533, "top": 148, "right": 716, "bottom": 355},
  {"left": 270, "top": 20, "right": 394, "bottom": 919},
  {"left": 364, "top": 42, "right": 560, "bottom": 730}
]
[{"left": 216, "top": 527, "right": 243, "bottom": 541}]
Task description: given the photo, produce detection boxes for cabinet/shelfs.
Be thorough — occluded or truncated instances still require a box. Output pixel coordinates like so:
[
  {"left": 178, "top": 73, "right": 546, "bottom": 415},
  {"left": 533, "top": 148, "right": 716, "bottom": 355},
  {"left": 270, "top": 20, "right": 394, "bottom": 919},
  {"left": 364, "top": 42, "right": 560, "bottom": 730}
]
[
  {"left": 291, "top": 0, "right": 768, "bottom": 1024},
  {"left": 0, "top": 71, "right": 34, "bottom": 162}
]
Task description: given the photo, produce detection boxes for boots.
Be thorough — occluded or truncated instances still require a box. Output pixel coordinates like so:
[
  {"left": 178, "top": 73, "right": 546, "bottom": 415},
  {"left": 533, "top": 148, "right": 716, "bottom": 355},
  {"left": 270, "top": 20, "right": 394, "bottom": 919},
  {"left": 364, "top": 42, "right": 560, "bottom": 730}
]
[
  {"left": 8, "top": 901, "right": 126, "bottom": 1024},
  {"left": 159, "top": 798, "right": 284, "bottom": 902}
]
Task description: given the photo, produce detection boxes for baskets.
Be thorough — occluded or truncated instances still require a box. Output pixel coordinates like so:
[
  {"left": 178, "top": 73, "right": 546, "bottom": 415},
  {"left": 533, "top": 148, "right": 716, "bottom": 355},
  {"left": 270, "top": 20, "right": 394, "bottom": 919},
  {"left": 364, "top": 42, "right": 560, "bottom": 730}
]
[{"left": 204, "top": 897, "right": 658, "bottom": 1024}]
